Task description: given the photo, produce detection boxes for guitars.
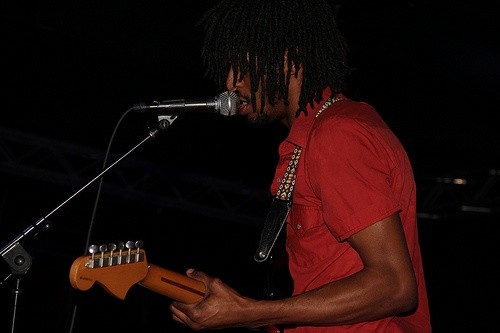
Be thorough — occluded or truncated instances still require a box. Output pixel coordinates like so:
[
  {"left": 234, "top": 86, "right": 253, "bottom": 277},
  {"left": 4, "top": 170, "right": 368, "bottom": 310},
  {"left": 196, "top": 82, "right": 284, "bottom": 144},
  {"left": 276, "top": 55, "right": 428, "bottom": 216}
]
[{"left": 69, "top": 241, "right": 282, "bottom": 333}]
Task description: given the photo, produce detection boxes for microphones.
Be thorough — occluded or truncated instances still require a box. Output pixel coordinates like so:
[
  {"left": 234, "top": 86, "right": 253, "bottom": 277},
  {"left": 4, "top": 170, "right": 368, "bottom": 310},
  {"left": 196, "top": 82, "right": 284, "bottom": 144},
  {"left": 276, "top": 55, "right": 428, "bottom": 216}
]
[{"left": 133, "top": 90, "right": 240, "bottom": 116}]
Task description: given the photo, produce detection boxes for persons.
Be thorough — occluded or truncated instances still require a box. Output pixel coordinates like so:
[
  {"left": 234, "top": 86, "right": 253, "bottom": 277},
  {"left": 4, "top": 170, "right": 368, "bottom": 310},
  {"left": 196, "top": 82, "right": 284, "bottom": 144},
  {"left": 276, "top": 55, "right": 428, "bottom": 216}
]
[{"left": 170, "top": 0, "right": 432, "bottom": 333}]
